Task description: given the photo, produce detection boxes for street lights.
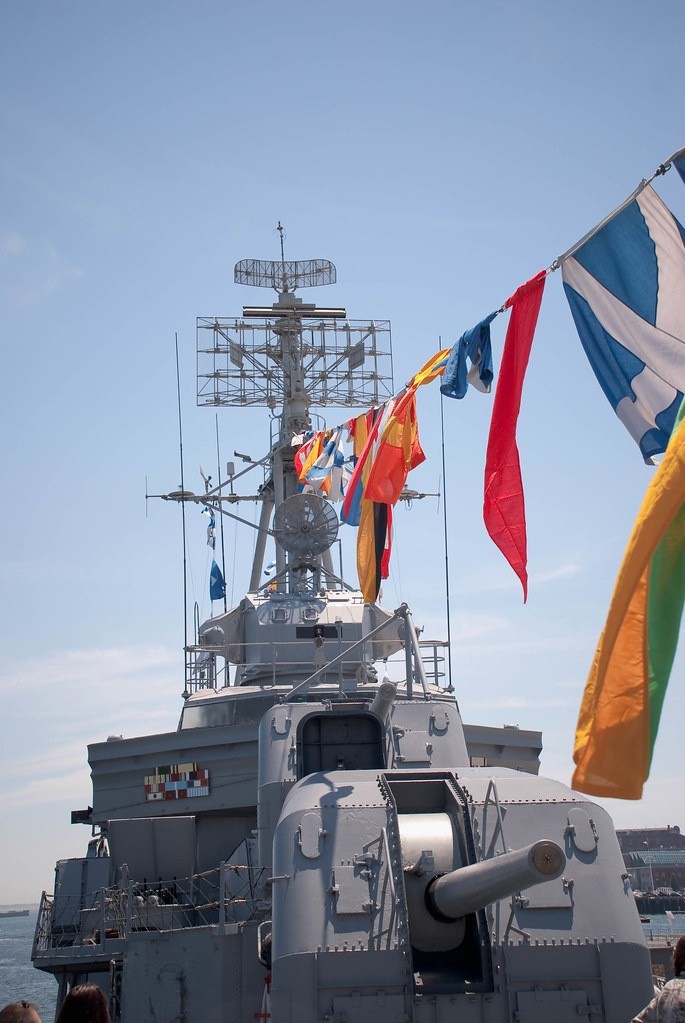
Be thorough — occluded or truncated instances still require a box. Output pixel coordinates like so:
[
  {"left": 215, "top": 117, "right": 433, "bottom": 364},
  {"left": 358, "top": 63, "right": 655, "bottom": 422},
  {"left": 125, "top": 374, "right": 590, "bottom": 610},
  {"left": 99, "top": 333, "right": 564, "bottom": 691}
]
[{"left": 643, "top": 842, "right": 654, "bottom": 894}]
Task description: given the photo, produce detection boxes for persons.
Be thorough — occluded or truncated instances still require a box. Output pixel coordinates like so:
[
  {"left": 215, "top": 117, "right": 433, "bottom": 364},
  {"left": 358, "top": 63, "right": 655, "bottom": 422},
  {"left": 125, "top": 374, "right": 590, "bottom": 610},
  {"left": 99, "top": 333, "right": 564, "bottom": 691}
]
[
  {"left": 0, "top": 1001, "right": 42, "bottom": 1023},
  {"left": 55, "top": 984, "right": 110, "bottom": 1023},
  {"left": 631, "top": 935, "right": 685, "bottom": 1023}
]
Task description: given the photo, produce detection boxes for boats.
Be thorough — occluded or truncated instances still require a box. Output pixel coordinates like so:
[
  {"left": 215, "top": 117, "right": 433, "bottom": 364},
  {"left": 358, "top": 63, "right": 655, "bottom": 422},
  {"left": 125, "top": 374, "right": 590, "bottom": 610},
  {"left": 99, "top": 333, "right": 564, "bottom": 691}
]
[{"left": 28, "top": 220, "right": 655, "bottom": 1023}]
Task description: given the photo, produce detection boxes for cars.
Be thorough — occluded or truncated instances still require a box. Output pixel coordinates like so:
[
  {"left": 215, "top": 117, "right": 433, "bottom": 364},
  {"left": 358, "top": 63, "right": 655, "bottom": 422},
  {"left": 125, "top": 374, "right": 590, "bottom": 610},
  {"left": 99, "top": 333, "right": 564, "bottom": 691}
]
[
  {"left": 640, "top": 892, "right": 657, "bottom": 897},
  {"left": 658, "top": 891, "right": 680, "bottom": 896}
]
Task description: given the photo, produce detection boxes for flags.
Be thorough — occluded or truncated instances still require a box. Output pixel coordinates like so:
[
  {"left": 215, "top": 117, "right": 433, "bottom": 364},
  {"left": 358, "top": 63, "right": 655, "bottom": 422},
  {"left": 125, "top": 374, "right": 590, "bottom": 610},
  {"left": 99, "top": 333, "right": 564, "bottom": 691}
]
[
  {"left": 209, "top": 561, "right": 227, "bottom": 600},
  {"left": 202, "top": 507, "right": 216, "bottom": 550},
  {"left": 560, "top": 183, "right": 684, "bottom": 465}
]
